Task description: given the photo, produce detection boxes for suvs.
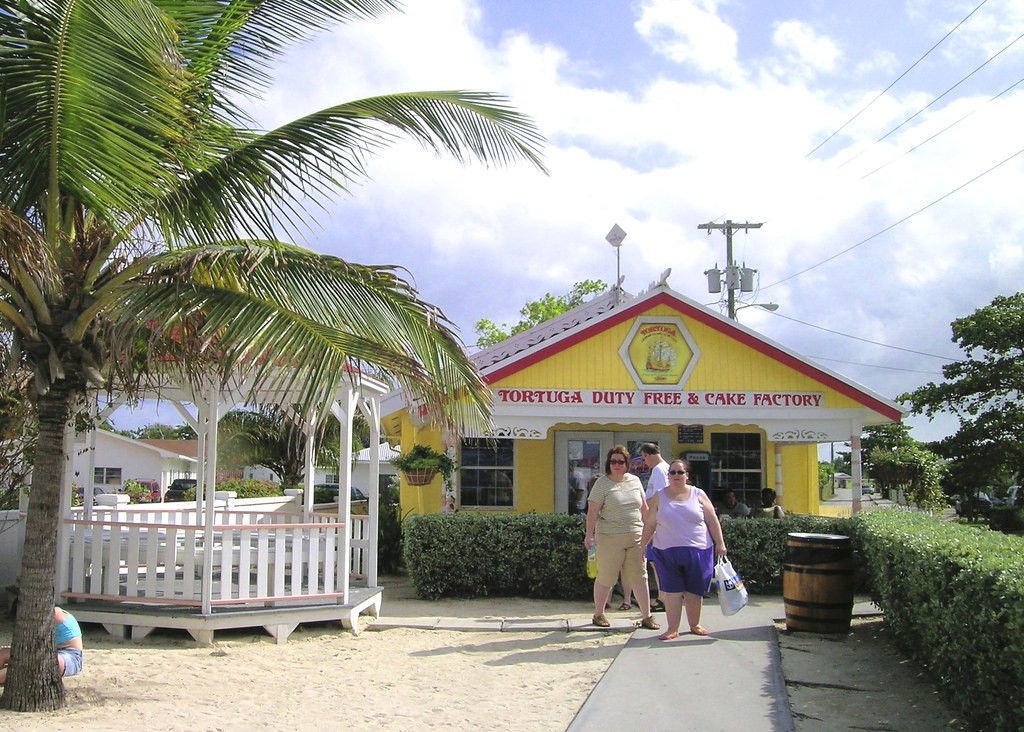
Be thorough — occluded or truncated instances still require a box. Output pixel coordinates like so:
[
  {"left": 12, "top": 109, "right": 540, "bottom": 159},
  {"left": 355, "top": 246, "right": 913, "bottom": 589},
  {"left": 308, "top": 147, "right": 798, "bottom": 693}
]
[
  {"left": 117, "top": 478, "right": 162, "bottom": 504},
  {"left": 164, "top": 479, "right": 198, "bottom": 502}
]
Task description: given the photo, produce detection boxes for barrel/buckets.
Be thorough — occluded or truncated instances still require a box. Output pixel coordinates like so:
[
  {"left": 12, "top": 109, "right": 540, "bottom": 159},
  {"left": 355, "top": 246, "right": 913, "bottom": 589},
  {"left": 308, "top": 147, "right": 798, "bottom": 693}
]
[{"left": 783, "top": 533, "right": 854, "bottom": 634}]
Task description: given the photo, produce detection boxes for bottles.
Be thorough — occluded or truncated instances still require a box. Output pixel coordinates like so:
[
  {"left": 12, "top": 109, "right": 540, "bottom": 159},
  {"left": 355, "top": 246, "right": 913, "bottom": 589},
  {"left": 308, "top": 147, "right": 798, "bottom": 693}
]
[{"left": 587, "top": 537, "right": 598, "bottom": 579}]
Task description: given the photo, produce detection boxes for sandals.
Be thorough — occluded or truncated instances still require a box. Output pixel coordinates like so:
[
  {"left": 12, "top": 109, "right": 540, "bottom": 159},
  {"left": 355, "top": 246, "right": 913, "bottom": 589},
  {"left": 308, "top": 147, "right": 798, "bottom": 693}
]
[
  {"left": 690, "top": 625, "right": 709, "bottom": 635},
  {"left": 659, "top": 630, "right": 679, "bottom": 640},
  {"left": 619, "top": 603, "right": 632, "bottom": 610},
  {"left": 651, "top": 599, "right": 666, "bottom": 612},
  {"left": 592, "top": 614, "right": 609, "bottom": 627},
  {"left": 605, "top": 602, "right": 610, "bottom": 608},
  {"left": 642, "top": 616, "right": 660, "bottom": 629}
]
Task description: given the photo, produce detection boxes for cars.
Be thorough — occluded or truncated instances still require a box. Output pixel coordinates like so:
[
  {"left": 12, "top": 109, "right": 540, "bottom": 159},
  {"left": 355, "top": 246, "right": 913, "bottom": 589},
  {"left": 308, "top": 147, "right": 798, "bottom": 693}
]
[
  {"left": 986, "top": 486, "right": 1024, "bottom": 507},
  {"left": 955, "top": 491, "right": 994, "bottom": 517},
  {"left": 301, "top": 483, "right": 367, "bottom": 506},
  {"left": 861, "top": 485, "right": 875, "bottom": 495},
  {"left": 76, "top": 485, "right": 105, "bottom": 508},
  {"left": 829, "top": 472, "right": 851, "bottom": 484}
]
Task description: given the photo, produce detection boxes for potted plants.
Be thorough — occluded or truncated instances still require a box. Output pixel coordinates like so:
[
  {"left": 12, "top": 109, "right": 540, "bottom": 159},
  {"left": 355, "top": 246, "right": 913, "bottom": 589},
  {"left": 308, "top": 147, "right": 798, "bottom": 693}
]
[{"left": 388, "top": 443, "right": 459, "bottom": 486}]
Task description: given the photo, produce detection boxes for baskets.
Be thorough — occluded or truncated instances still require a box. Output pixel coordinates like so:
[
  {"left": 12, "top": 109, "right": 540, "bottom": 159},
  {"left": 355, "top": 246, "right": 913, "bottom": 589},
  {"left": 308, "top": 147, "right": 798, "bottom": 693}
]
[{"left": 401, "top": 466, "right": 438, "bottom": 486}]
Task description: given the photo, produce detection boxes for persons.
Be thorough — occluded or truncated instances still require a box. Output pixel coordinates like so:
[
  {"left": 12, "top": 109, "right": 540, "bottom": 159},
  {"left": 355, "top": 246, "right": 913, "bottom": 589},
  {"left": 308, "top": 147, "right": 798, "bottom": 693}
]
[
  {"left": 584, "top": 445, "right": 661, "bottom": 629},
  {"left": 0, "top": 599, "right": 83, "bottom": 687},
  {"left": 588, "top": 442, "right": 669, "bottom": 612},
  {"left": 717, "top": 487, "right": 784, "bottom": 519},
  {"left": 480, "top": 459, "right": 513, "bottom": 506},
  {"left": 641, "top": 460, "right": 727, "bottom": 640},
  {"left": 569, "top": 460, "right": 585, "bottom": 514}
]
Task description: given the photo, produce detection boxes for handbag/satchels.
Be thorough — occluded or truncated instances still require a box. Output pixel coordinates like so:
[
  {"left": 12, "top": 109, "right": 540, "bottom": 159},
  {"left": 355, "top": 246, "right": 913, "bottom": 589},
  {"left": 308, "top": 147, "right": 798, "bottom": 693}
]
[{"left": 714, "top": 554, "right": 749, "bottom": 616}]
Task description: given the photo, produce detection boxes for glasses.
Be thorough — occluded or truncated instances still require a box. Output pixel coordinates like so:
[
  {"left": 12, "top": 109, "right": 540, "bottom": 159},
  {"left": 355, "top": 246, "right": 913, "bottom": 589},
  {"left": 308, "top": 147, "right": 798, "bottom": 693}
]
[
  {"left": 609, "top": 459, "right": 625, "bottom": 465},
  {"left": 669, "top": 470, "right": 686, "bottom": 475}
]
